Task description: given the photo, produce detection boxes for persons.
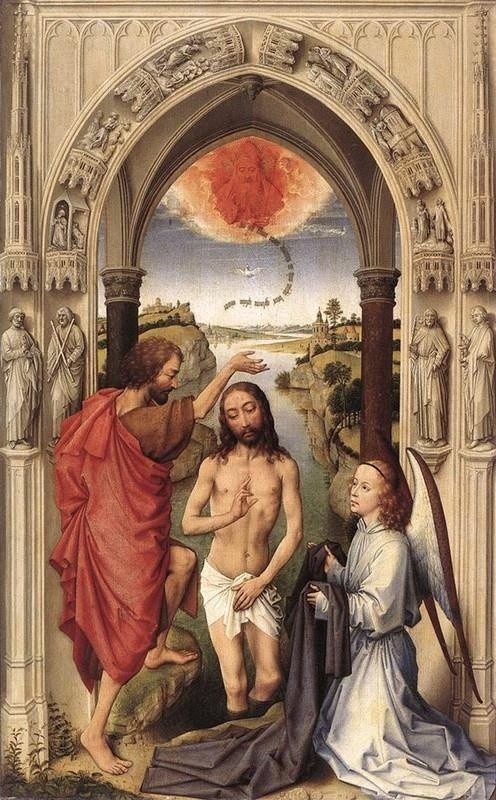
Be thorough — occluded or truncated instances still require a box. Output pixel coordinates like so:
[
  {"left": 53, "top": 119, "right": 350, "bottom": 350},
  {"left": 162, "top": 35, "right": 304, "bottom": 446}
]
[{"left": 0, "top": 306, "right": 496, "bottom": 775}]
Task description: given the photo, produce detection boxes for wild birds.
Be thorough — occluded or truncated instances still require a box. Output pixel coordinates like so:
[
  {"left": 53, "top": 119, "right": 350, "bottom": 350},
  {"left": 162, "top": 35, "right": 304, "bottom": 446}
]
[{"left": 229, "top": 264, "right": 265, "bottom": 278}]
